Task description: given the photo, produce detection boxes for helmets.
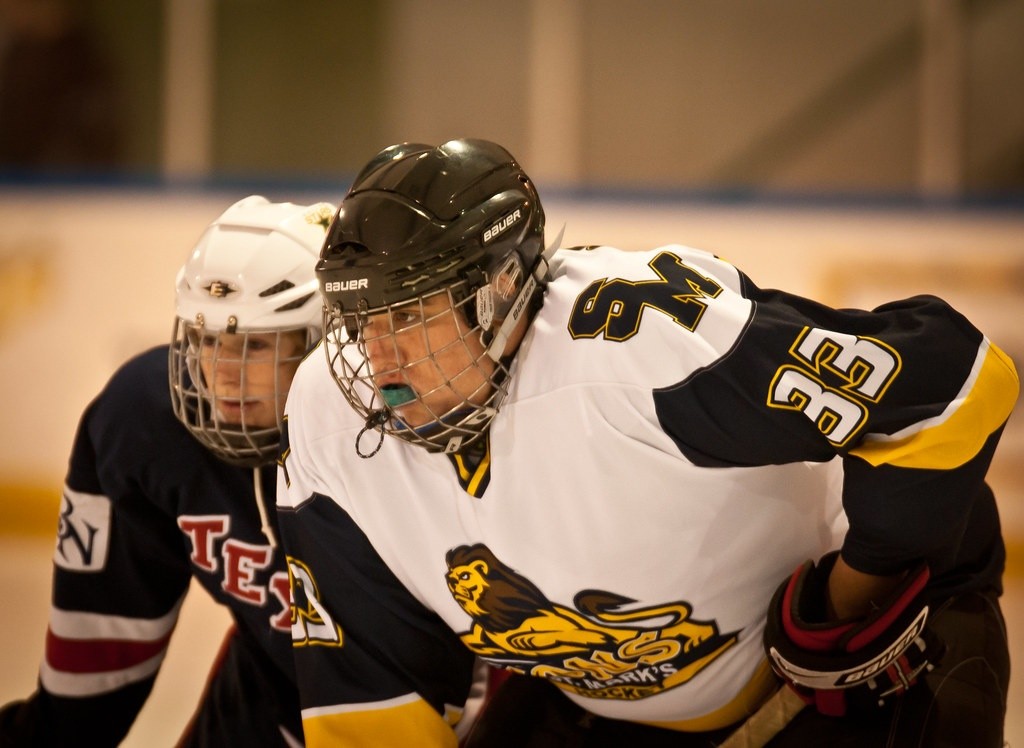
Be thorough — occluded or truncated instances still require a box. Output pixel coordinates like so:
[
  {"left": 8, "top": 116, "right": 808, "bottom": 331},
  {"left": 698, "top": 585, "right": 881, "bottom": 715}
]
[
  {"left": 165, "top": 196, "right": 346, "bottom": 466},
  {"left": 317, "top": 137, "right": 547, "bottom": 457}
]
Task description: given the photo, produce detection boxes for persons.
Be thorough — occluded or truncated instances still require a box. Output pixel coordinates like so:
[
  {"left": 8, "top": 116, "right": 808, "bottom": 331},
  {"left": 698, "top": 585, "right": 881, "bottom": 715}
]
[
  {"left": 1, "top": 196, "right": 421, "bottom": 747},
  {"left": 275, "top": 140, "right": 1021, "bottom": 748}
]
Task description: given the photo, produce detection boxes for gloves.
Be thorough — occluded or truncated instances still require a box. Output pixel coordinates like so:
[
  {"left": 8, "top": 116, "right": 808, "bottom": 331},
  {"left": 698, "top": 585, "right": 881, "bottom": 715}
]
[{"left": 762, "top": 551, "right": 943, "bottom": 716}]
